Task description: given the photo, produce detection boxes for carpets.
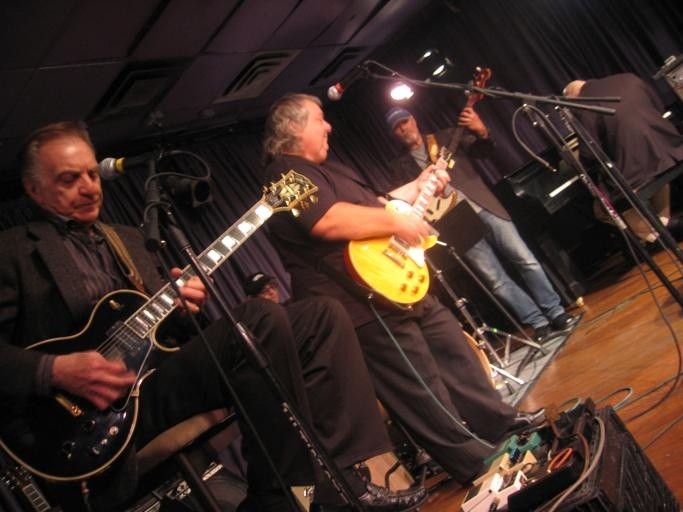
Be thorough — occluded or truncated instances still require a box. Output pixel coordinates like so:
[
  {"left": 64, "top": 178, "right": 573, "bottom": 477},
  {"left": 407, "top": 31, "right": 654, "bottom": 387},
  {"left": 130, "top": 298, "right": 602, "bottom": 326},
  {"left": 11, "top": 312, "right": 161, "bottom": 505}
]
[{"left": 489, "top": 310, "right": 588, "bottom": 412}]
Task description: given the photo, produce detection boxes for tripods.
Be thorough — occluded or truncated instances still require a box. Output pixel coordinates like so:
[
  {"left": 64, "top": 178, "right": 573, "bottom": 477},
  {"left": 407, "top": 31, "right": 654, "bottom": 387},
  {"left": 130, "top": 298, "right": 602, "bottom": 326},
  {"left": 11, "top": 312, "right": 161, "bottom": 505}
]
[
  {"left": 425, "top": 253, "right": 548, "bottom": 393},
  {"left": 442, "top": 239, "right": 571, "bottom": 377}
]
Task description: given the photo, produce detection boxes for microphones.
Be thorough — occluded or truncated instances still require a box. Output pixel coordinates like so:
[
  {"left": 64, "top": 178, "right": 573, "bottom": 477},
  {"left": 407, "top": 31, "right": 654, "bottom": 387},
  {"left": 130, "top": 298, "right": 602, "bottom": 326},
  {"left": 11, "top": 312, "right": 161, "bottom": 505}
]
[
  {"left": 99, "top": 149, "right": 160, "bottom": 181},
  {"left": 327, "top": 62, "right": 369, "bottom": 102}
]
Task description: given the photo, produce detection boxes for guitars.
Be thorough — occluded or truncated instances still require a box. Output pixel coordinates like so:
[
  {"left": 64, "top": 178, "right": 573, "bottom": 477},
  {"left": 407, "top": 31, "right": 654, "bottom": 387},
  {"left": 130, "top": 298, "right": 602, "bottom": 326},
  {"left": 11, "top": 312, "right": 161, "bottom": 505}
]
[
  {"left": 344, "top": 66, "right": 491, "bottom": 312},
  {"left": 1, "top": 170, "right": 319, "bottom": 481}
]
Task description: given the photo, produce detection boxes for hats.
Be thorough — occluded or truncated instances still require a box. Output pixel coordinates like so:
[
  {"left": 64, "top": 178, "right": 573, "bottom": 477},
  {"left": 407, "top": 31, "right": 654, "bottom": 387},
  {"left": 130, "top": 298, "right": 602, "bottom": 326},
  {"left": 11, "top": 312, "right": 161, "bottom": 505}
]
[
  {"left": 383, "top": 107, "right": 410, "bottom": 136},
  {"left": 244, "top": 272, "right": 276, "bottom": 297}
]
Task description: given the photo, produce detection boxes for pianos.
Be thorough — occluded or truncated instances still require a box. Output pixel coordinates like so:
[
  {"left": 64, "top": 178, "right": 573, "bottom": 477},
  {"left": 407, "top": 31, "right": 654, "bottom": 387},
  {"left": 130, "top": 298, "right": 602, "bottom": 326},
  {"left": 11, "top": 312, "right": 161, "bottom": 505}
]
[{"left": 491, "top": 101, "right": 682, "bottom": 307}]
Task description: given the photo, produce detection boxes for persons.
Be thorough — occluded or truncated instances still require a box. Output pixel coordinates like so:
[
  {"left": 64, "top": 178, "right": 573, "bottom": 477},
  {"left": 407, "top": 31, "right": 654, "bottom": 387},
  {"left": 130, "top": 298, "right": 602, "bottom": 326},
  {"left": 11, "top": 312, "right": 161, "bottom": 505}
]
[
  {"left": 261, "top": 88, "right": 552, "bottom": 489},
  {"left": 385, "top": 102, "right": 578, "bottom": 342},
  {"left": 558, "top": 71, "right": 682, "bottom": 258},
  {"left": 1, "top": 119, "right": 431, "bottom": 511},
  {"left": 244, "top": 269, "right": 280, "bottom": 309}
]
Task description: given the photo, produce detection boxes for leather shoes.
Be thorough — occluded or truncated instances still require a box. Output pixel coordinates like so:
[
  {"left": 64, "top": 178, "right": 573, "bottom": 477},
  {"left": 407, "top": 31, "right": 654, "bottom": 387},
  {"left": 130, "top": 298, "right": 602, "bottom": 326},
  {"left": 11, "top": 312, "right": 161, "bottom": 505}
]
[
  {"left": 535, "top": 328, "right": 546, "bottom": 342},
  {"left": 554, "top": 313, "right": 574, "bottom": 330},
  {"left": 504, "top": 408, "right": 545, "bottom": 434},
  {"left": 310, "top": 481, "right": 428, "bottom": 511}
]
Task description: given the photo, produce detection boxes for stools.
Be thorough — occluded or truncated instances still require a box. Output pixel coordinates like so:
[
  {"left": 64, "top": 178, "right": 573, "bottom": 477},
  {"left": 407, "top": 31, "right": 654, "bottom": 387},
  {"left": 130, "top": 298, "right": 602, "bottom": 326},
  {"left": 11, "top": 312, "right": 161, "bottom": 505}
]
[
  {"left": 127, "top": 408, "right": 271, "bottom": 509},
  {"left": 611, "top": 157, "right": 682, "bottom": 261}
]
